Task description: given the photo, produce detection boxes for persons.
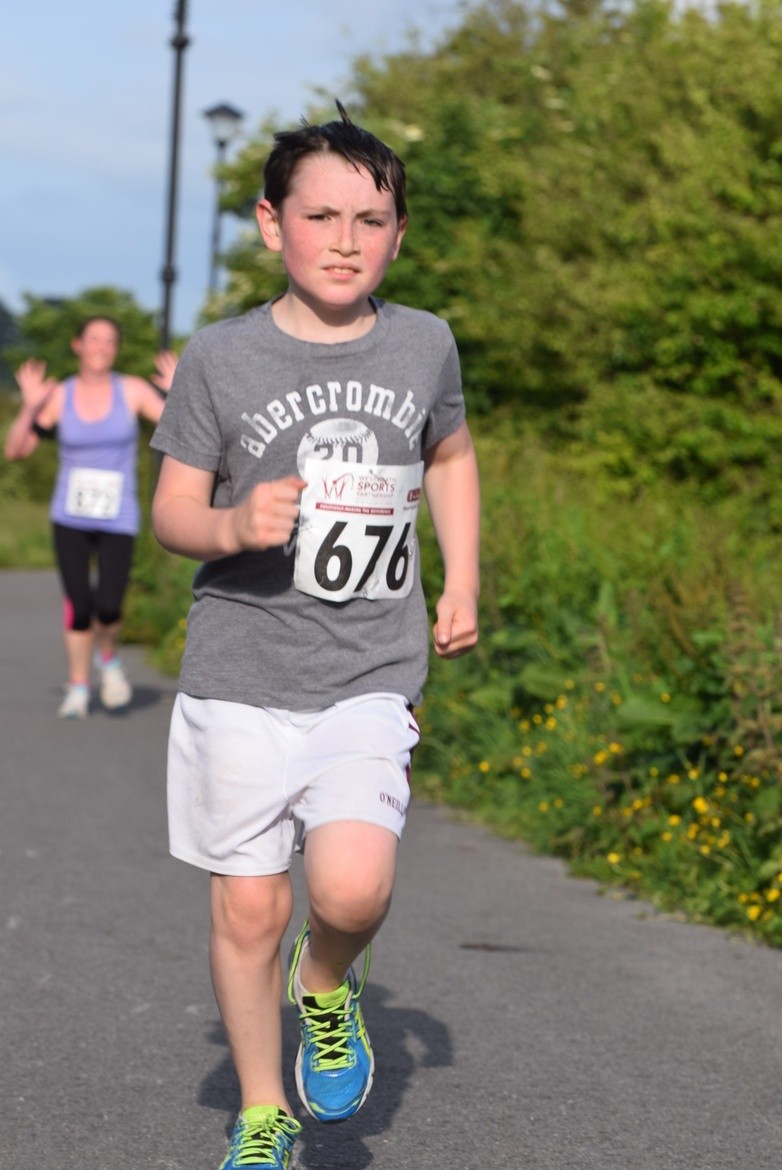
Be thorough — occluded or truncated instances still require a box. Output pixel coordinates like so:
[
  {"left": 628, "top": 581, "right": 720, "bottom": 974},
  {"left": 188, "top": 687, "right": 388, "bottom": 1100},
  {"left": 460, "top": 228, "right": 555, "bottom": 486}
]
[
  {"left": 149, "top": 99, "right": 482, "bottom": 1170},
  {"left": 6, "top": 318, "right": 180, "bottom": 719}
]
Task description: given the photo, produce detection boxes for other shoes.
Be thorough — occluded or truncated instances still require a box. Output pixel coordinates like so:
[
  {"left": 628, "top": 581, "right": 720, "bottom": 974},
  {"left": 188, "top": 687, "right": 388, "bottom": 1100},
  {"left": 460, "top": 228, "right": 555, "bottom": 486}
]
[
  {"left": 59, "top": 688, "right": 92, "bottom": 719},
  {"left": 90, "top": 649, "right": 133, "bottom": 709}
]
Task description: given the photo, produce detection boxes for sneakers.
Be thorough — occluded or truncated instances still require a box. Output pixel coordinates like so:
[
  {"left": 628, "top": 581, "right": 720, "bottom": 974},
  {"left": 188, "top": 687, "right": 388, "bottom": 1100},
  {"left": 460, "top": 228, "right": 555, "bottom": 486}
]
[
  {"left": 286, "top": 910, "right": 377, "bottom": 1125},
  {"left": 216, "top": 1105, "right": 308, "bottom": 1170}
]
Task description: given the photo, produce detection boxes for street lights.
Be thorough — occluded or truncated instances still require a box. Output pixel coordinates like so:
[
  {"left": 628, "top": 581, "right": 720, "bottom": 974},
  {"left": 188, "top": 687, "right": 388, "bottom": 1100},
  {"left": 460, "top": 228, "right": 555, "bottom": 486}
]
[{"left": 204, "top": 98, "right": 247, "bottom": 298}]
